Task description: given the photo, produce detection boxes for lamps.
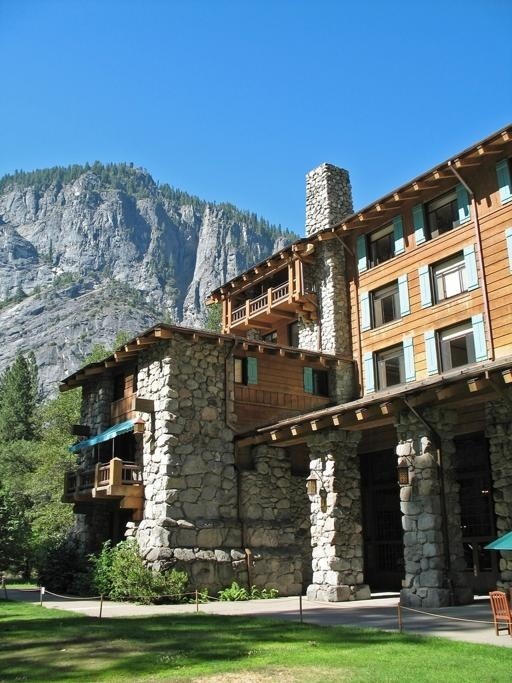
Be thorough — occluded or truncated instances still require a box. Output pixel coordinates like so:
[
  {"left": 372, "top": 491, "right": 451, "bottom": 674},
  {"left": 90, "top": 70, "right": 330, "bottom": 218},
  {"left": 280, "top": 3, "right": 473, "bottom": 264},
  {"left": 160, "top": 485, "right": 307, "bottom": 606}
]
[
  {"left": 132, "top": 416, "right": 146, "bottom": 434},
  {"left": 306, "top": 468, "right": 323, "bottom": 496},
  {"left": 396, "top": 454, "right": 416, "bottom": 484}
]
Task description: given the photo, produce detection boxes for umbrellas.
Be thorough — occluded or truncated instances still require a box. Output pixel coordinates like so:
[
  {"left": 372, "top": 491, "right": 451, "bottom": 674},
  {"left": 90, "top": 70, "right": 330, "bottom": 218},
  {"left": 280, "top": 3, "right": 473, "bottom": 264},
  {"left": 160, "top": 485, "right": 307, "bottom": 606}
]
[{"left": 482, "top": 530, "right": 512, "bottom": 550}]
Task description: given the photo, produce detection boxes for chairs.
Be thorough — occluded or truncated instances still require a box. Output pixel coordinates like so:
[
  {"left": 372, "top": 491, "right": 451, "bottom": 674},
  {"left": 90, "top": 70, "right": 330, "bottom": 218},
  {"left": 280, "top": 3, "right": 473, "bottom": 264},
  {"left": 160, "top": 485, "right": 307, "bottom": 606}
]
[{"left": 488, "top": 590, "right": 512, "bottom": 636}]
